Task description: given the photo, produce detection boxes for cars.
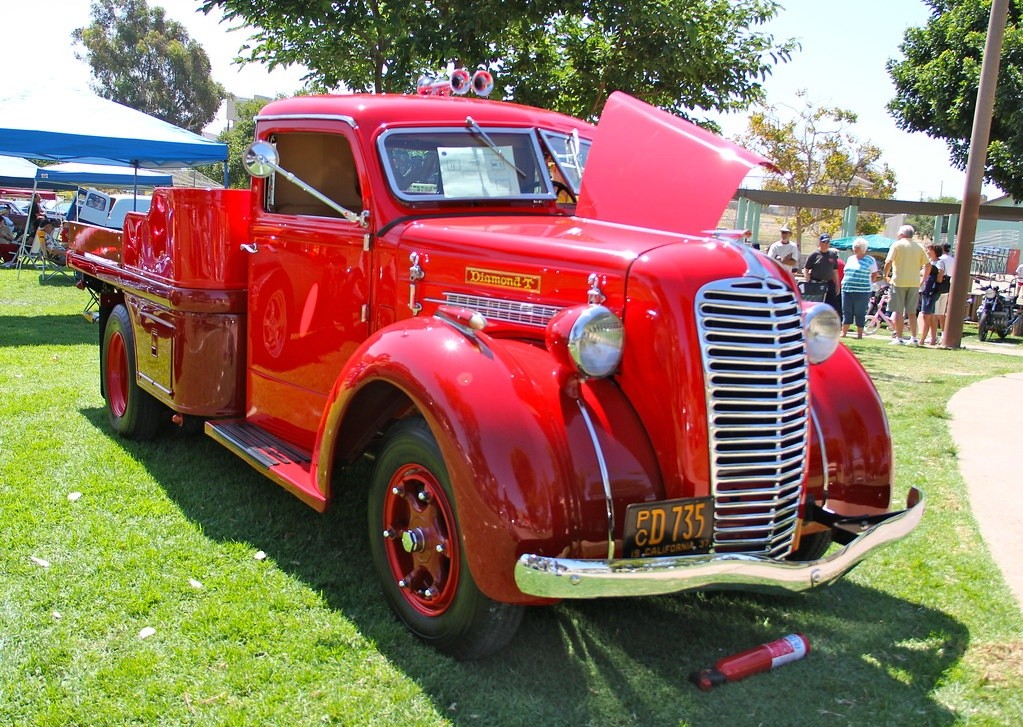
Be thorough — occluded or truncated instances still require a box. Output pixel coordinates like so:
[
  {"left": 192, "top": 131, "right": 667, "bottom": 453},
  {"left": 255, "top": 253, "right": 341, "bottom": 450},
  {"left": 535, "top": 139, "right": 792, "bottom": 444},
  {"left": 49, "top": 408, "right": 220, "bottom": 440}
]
[
  {"left": 67, "top": 69, "right": 927, "bottom": 659},
  {"left": 44, "top": 199, "right": 83, "bottom": 225}
]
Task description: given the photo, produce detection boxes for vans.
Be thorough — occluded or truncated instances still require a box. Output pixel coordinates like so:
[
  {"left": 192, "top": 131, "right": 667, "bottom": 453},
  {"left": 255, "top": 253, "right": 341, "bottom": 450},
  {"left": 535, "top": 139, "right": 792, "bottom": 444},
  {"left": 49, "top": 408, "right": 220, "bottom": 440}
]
[{"left": 64, "top": 187, "right": 152, "bottom": 231}]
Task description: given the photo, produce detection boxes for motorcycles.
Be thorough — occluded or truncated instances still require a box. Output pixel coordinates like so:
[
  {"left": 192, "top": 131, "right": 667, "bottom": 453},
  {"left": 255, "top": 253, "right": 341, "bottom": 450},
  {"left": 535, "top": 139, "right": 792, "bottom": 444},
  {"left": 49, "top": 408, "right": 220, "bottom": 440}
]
[{"left": 974, "top": 278, "right": 1023, "bottom": 342}]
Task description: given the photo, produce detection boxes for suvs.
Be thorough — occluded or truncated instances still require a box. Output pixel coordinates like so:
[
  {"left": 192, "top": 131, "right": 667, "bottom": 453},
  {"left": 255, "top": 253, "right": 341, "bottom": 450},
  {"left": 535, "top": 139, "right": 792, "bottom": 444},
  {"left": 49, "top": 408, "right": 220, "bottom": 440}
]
[{"left": 0, "top": 200, "right": 30, "bottom": 239}]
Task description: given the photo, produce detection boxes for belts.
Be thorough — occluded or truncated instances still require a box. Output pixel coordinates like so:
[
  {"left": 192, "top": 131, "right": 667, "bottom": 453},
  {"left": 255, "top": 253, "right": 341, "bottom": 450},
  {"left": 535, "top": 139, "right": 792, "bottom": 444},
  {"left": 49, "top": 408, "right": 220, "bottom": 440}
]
[{"left": 813, "top": 278, "right": 833, "bottom": 283}]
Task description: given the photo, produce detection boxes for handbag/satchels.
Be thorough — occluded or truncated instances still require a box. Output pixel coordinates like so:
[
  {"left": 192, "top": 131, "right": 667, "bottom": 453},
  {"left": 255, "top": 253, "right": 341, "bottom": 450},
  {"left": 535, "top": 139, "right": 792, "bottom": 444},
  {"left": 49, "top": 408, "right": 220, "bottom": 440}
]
[{"left": 936, "top": 263, "right": 951, "bottom": 293}]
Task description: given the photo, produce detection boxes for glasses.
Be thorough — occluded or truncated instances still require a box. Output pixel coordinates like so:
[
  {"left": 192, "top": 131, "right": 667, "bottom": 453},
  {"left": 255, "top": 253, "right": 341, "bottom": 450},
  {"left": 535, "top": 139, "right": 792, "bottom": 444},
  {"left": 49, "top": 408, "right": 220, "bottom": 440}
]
[{"left": 781, "top": 231, "right": 790, "bottom": 234}]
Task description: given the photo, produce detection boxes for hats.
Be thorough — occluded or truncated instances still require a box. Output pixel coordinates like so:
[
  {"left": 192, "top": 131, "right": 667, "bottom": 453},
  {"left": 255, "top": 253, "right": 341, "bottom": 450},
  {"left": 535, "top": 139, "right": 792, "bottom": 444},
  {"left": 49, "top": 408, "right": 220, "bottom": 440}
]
[
  {"left": 779, "top": 226, "right": 791, "bottom": 232},
  {"left": 819, "top": 233, "right": 830, "bottom": 242}
]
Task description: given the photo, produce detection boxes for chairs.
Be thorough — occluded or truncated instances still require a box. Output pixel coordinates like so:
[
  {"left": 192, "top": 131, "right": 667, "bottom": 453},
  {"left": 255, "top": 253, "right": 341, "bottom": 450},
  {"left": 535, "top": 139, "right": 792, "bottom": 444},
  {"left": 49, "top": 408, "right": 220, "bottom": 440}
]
[
  {"left": 5, "top": 227, "right": 61, "bottom": 268},
  {"left": 75, "top": 271, "right": 100, "bottom": 313},
  {"left": 37, "top": 230, "right": 73, "bottom": 282},
  {"left": 796, "top": 282, "right": 829, "bottom": 302}
]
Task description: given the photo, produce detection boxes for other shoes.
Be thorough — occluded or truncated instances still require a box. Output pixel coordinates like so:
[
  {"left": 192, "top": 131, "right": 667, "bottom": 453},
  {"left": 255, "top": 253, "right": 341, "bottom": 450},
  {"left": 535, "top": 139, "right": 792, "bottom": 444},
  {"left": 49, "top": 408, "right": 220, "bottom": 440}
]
[
  {"left": 888, "top": 338, "right": 904, "bottom": 345},
  {"left": 905, "top": 340, "right": 918, "bottom": 347}
]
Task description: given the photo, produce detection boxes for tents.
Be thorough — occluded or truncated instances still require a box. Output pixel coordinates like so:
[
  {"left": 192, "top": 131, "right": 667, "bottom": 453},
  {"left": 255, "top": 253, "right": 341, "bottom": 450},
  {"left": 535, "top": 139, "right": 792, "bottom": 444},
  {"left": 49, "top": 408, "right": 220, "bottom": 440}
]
[
  {"left": 0, "top": 154, "right": 79, "bottom": 250},
  {"left": 18, "top": 162, "right": 173, "bottom": 238},
  {"left": 0, "top": 82, "right": 230, "bottom": 190},
  {"left": 829, "top": 235, "right": 897, "bottom": 252}
]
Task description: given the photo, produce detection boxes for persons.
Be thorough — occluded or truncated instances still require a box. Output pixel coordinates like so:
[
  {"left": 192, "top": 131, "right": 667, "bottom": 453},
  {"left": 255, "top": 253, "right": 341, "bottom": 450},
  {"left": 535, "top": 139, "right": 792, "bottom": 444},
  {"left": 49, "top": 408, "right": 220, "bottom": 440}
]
[
  {"left": 28, "top": 194, "right": 45, "bottom": 234},
  {"left": 882, "top": 295, "right": 906, "bottom": 338},
  {"left": 803, "top": 233, "right": 840, "bottom": 312},
  {"left": 0, "top": 216, "right": 18, "bottom": 262},
  {"left": 935, "top": 243, "right": 953, "bottom": 335},
  {"left": 918, "top": 244, "right": 947, "bottom": 347},
  {"left": 768, "top": 224, "right": 799, "bottom": 274},
  {"left": 840, "top": 238, "right": 878, "bottom": 339},
  {"left": 883, "top": 225, "right": 932, "bottom": 346},
  {"left": 29, "top": 218, "right": 82, "bottom": 281},
  {"left": 1015, "top": 265, "right": 1023, "bottom": 297}
]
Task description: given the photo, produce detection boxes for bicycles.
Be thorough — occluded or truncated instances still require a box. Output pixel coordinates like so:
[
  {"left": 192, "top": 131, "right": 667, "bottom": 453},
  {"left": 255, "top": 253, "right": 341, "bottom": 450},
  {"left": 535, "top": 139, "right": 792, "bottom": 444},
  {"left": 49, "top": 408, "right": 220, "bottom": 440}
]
[{"left": 863, "top": 276, "right": 918, "bottom": 339}]
